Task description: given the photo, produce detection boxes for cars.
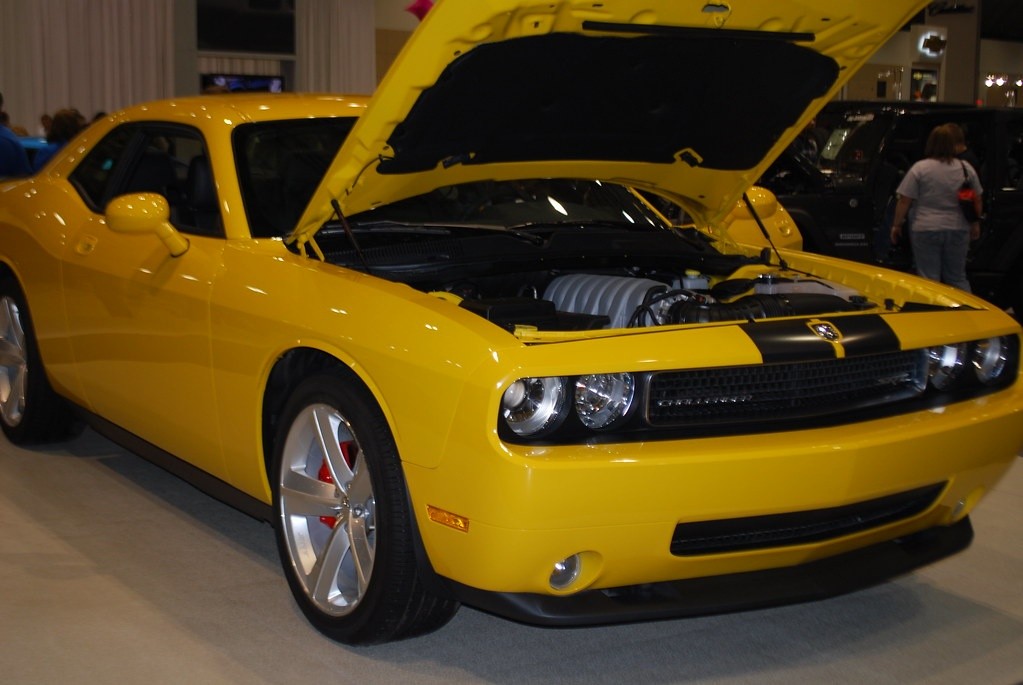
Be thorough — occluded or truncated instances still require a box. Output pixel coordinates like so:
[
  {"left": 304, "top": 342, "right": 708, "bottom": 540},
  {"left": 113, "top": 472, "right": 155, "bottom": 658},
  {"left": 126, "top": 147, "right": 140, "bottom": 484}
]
[{"left": 0, "top": 0, "right": 1023, "bottom": 646}]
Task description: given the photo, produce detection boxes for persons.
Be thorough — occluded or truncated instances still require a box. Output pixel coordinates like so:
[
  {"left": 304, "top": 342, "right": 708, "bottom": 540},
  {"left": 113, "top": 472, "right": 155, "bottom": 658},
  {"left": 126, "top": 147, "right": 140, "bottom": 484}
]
[
  {"left": 0, "top": 94, "right": 113, "bottom": 175},
  {"left": 887, "top": 122, "right": 997, "bottom": 297}
]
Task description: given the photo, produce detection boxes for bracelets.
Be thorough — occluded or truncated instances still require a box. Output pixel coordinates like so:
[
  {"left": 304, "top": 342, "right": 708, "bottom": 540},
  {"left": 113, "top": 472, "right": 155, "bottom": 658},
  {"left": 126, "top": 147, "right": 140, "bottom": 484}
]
[{"left": 893, "top": 222, "right": 901, "bottom": 227}]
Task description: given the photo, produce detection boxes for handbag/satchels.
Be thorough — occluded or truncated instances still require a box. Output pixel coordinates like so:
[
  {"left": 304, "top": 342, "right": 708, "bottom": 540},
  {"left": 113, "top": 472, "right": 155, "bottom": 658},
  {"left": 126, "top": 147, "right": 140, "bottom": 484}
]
[{"left": 958, "top": 159, "right": 978, "bottom": 224}]
